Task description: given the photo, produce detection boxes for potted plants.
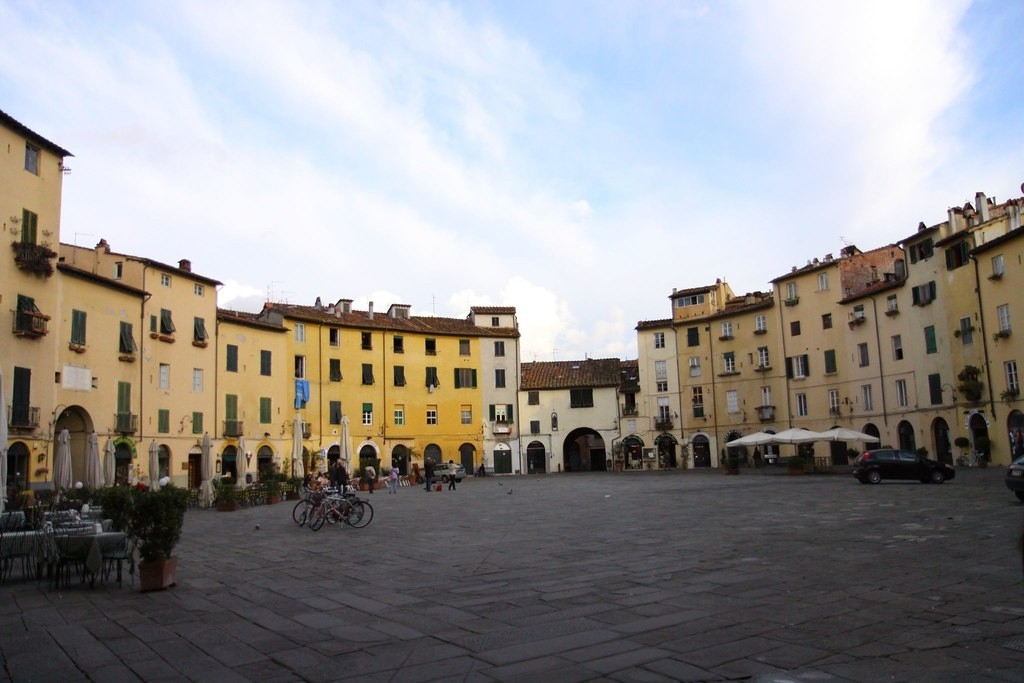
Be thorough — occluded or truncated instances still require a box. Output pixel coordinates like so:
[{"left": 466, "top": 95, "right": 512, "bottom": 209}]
[
  {"left": 974, "top": 436, "right": 991, "bottom": 468},
  {"left": 783, "top": 456, "right": 804, "bottom": 475},
  {"left": 127, "top": 484, "right": 191, "bottom": 591},
  {"left": 614, "top": 442, "right": 624, "bottom": 472},
  {"left": 355, "top": 466, "right": 379, "bottom": 490},
  {"left": 212, "top": 479, "right": 238, "bottom": 510},
  {"left": 402, "top": 446, "right": 421, "bottom": 485},
  {"left": 286, "top": 479, "right": 299, "bottom": 499},
  {"left": 263, "top": 462, "right": 280, "bottom": 504},
  {"left": 721, "top": 456, "right": 740, "bottom": 475}
]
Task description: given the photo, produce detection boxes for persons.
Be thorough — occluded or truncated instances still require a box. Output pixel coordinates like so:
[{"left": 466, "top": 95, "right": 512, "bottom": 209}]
[
  {"left": 424, "top": 457, "right": 436, "bottom": 492},
  {"left": 388, "top": 463, "right": 399, "bottom": 495},
  {"left": 303, "top": 462, "right": 351, "bottom": 495},
  {"left": 365, "top": 462, "right": 377, "bottom": 493},
  {"left": 447, "top": 460, "right": 461, "bottom": 491},
  {"left": 662, "top": 452, "right": 672, "bottom": 471}
]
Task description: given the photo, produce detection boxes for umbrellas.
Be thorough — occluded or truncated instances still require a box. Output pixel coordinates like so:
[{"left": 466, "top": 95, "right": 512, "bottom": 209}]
[
  {"left": 724, "top": 427, "right": 880, "bottom": 473},
  {"left": 101, "top": 435, "right": 117, "bottom": 489},
  {"left": 52, "top": 427, "right": 76, "bottom": 491},
  {"left": 338, "top": 414, "right": 354, "bottom": 479},
  {"left": 146, "top": 438, "right": 163, "bottom": 492},
  {"left": 196, "top": 430, "right": 217, "bottom": 510},
  {"left": 83, "top": 429, "right": 101, "bottom": 489},
  {"left": 234, "top": 435, "right": 249, "bottom": 490},
  {"left": 291, "top": 412, "right": 305, "bottom": 488}
]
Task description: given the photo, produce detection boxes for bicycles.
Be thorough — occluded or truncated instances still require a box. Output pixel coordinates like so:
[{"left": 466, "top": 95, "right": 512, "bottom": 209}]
[{"left": 292, "top": 486, "right": 374, "bottom": 531}]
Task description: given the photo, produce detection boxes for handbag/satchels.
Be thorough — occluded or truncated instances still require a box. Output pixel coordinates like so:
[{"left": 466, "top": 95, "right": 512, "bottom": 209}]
[
  {"left": 369, "top": 474, "right": 375, "bottom": 479},
  {"left": 436, "top": 485, "right": 442, "bottom": 491}
]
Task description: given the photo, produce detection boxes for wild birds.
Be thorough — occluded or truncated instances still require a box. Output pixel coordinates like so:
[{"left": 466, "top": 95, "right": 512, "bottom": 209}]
[
  {"left": 507, "top": 489, "right": 512, "bottom": 494},
  {"left": 498, "top": 482, "right": 503, "bottom": 485}
]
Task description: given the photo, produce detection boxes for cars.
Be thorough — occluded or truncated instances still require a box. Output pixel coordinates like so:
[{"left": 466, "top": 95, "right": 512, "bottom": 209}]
[
  {"left": 851, "top": 449, "right": 955, "bottom": 484},
  {"left": 1005, "top": 455, "right": 1024, "bottom": 501},
  {"left": 412, "top": 463, "right": 466, "bottom": 484}
]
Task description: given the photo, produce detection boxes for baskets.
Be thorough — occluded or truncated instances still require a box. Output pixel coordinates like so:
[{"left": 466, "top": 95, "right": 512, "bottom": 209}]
[{"left": 299, "top": 488, "right": 311, "bottom": 500}]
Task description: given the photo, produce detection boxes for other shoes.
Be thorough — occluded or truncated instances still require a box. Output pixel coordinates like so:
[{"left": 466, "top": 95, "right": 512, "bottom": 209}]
[{"left": 427, "top": 489, "right": 432, "bottom": 492}]
